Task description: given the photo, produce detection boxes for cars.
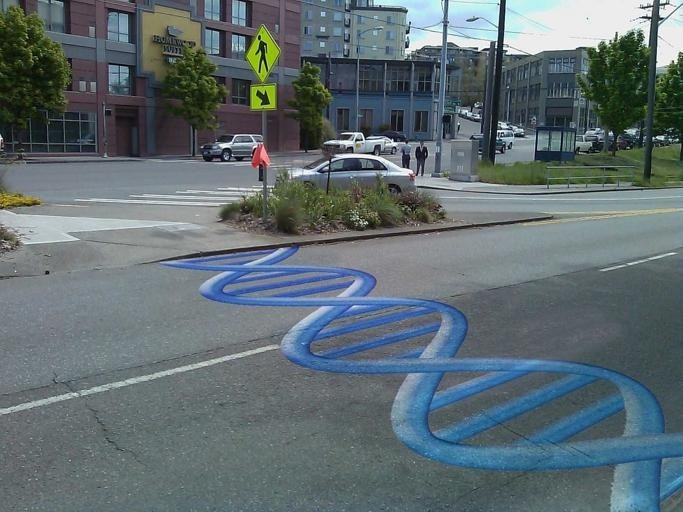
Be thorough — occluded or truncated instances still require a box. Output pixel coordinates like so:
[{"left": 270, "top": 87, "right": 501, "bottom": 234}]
[
  {"left": 458, "top": 110, "right": 482, "bottom": 122},
  {"left": 366, "top": 131, "right": 406, "bottom": 154},
  {"left": 276, "top": 154, "right": 417, "bottom": 194},
  {"left": 498, "top": 121, "right": 525, "bottom": 137},
  {"left": 469, "top": 133, "right": 506, "bottom": 153},
  {"left": 559, "top": 126, "right": 679, "bottom": 160}
]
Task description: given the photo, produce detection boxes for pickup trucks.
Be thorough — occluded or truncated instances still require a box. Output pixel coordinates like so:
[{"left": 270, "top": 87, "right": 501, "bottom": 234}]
[{"left": 323, "top": 132, "right": 385, "bottom": 155}]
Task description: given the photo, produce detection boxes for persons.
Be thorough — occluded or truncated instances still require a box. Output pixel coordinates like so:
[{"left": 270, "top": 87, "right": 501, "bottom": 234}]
[
  {"left": 400, "top": 139, "right": 411, "bottom": 168},
  {"left": 415, "top": 140, "right": 428, "bottom": 176},
  {"left": 458, "top": 121, "right": 460, "bottom": 131}
]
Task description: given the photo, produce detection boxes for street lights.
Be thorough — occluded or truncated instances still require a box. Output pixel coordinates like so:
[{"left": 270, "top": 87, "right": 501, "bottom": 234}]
[
  {"left": 354, "top": 26, "right": 384, "bottom": 132},
  {"left": 466, "top": 16, "right": 505, "bottom": 166}
]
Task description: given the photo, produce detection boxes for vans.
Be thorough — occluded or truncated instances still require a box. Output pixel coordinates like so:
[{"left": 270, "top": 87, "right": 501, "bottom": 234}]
[{"left": 496, "top": 129, "right": 514, "bottom": 149}]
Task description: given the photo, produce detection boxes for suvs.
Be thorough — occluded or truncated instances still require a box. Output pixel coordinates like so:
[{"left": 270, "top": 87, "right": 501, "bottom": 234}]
[{"left": 200, "top": 134, "right": 264, "bottom": 162}]
